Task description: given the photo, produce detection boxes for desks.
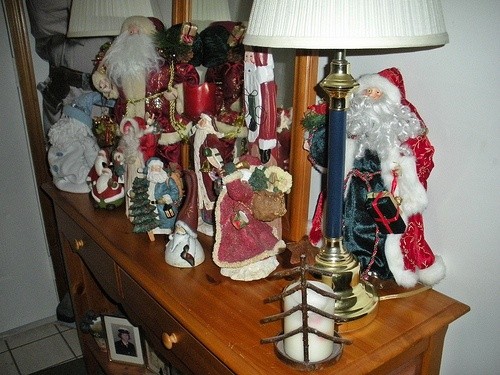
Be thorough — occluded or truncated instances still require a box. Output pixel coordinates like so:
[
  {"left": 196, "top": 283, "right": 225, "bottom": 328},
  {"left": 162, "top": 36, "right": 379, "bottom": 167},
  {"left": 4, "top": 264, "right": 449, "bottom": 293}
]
[{"left": 43, "top": 185, "right": 471, "bottom": 375}]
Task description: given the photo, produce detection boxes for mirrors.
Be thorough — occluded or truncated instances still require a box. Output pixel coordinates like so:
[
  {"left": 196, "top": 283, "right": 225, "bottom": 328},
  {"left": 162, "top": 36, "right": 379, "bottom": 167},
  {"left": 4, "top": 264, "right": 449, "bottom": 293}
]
[{"left": 173, "top": 0, "right": 301, "bottom": 242}]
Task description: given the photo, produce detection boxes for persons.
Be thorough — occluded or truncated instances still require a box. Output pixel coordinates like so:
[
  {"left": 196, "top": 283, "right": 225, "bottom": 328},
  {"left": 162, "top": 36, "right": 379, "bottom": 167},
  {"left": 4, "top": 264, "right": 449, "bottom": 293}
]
[
  {"left": 113, "top": 328, "right": 137, "bottom": 357},
  {"left": 44, "top": 15, "right": 447, "bottom": 288}
]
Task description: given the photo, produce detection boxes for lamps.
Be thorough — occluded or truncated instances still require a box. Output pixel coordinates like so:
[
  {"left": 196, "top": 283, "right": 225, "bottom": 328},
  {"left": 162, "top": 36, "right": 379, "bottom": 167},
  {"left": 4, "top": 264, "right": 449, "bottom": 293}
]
[
  {"left": 239, "top": 0, "right": 450, "bottom": 336},
  {"left": 66, "top": 0, "right": 156, "bottom": 37},
  {"left": 256, "top": 233, "right": 355, "bottom": 373}
]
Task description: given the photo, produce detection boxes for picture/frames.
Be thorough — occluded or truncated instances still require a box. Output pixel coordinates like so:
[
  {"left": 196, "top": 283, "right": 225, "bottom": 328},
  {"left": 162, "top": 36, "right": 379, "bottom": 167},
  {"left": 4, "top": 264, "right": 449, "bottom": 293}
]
[{"left": 101, "top": 314, "right": 148, "bottom": 368}]
